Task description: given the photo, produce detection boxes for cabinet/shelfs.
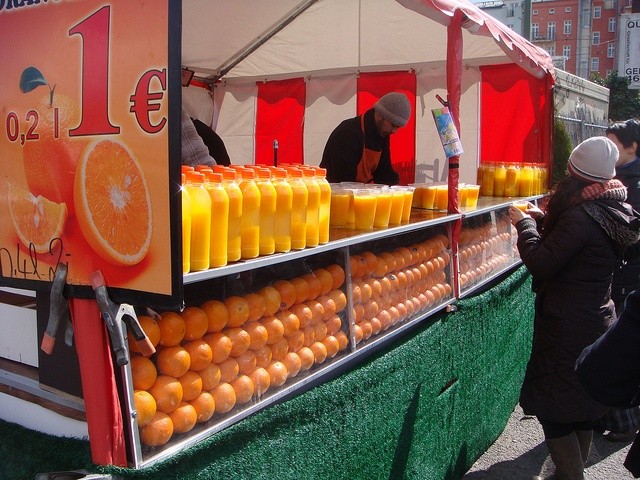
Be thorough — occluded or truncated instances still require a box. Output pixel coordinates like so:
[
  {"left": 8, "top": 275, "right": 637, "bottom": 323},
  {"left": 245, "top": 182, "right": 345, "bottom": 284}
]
[
  {"left": 114, "top": 194, "right": 545, "bottom": 474},
  {"left": 0, "top": 284, "right": 89, "bottom": 442}
]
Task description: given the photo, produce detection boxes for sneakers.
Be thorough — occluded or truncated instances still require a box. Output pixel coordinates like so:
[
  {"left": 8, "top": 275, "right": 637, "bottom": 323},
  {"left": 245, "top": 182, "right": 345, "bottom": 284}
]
[
  {"left": 607, "top": 429, "right": 637, "bottom": 442},
  {"left": 594, "top": 431, "right": 603, "bottom": 438}
]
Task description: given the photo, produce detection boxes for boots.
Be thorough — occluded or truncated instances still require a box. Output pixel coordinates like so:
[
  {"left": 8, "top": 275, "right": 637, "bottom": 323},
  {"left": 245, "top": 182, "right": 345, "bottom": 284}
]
[
  {"left": 532, "top": 432, "right": 586, "bottom": 480},
  {"left": 573, "top": 431, "right": 592, "bottom": 465}
]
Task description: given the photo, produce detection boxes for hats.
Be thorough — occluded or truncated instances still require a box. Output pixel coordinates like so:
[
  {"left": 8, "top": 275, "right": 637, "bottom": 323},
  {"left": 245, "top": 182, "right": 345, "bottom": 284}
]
[
  {"left": 566, "top": 135, "right": 619, "bottom": 184},
  {"left": 375, "top": 92, "right": 410, "bottom": 127}
]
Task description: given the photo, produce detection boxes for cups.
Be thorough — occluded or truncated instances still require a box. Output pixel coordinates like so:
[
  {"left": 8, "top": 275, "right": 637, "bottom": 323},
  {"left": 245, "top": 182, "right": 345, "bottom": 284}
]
[
  {"left": 354, "top": 189, "right": 378, "bottom": 230},
  {"left": 422, "top": 187, "right": 436, "bottom": 208},
  {"left": 391, "top": 186, "right": 416, "bottom": 222},
  {"left": 513, "top": 201, "right": 527, "bottom": 211},
  {"left": 459, "top": 185, "right": 468, "bottom": 207},
  {"left": 330, "top": 188, "right": 351, "bottom": 226},
  {"left": 376, "top": 189, "right": 395, "bottom": 226},
  {"left": 341, "top": 182, "right": 364, "bottom": 188},
  {"left": 408, "top": 184, "right": 426, "bottom": 208},
  {"left": 468, "top": 185, "right": 480, "bottom": 206},
  {"left": 365, "top": 183, "right": 388, "bottom": 188},
  {"left": 437, "top": 187, "right": 448, "bottom": 209},
  {"left": 390, "top": 188, "right": 407, "bottom": 225}
]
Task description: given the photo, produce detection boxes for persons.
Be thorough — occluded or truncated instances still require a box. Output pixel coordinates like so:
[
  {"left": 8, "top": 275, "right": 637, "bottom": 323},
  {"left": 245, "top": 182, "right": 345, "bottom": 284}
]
[
  {"left": 319, "top": 93, "right": 410, "bottom": 187},
  {"left": 604, "top": 119, "right": 640, "bottom": 442},
  {"left": 508, "top": 137, "right": 640, "bottom": 480}
]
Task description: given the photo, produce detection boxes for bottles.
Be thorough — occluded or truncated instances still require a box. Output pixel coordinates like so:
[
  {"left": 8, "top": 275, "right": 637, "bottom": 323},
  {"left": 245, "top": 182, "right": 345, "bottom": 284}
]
[
  {"left": 183, "top": 163, "right": 330, "bottom": 274},
  {"left": 476, "top": 158, "right": 548, "bottom": 197}
]
